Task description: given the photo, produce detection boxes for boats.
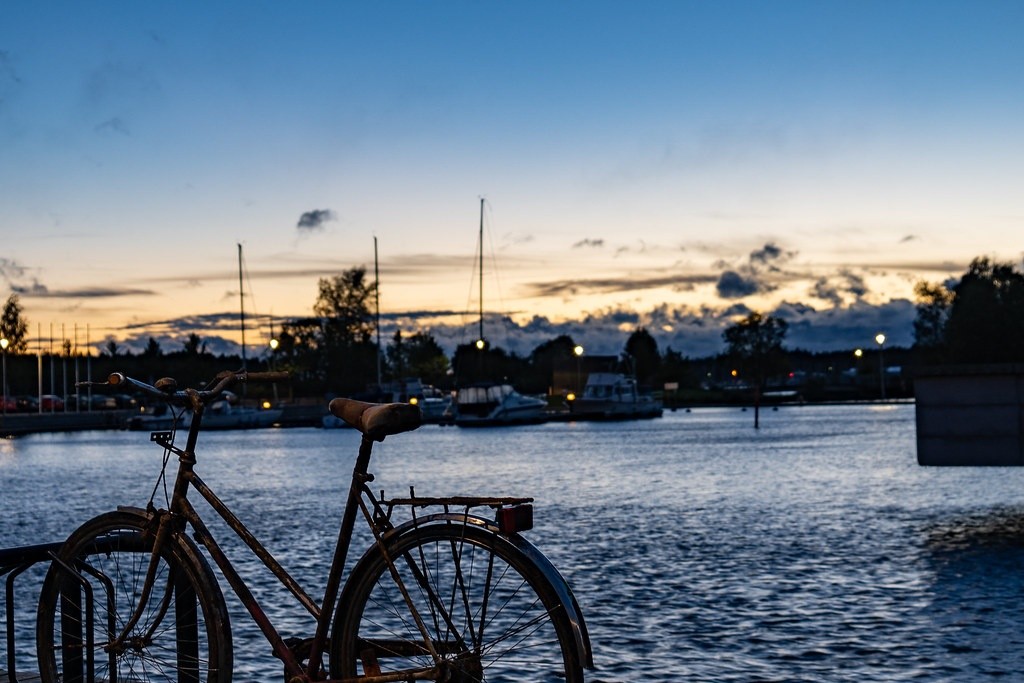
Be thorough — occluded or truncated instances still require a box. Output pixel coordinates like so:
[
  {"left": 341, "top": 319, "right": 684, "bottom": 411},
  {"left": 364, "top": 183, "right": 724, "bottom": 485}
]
[
  {"left": 564, "top": 371, "right": 664, "bottom": 419},
  {"left": 127, "top": 391, "right": 238, "bottom": 432}
]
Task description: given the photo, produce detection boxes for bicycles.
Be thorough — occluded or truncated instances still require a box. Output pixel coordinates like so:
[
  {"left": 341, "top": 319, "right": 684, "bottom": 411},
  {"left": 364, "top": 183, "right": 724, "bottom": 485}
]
[{"left": 35, "top": 370, "right": 597, "bottom": 683}]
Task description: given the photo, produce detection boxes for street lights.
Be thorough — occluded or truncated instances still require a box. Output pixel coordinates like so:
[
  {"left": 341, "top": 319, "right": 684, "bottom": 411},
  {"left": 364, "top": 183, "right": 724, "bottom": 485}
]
[
  {"left": 855, "top": 349, "right": 862, "bottom": 402},
  {"left": 269, "top": 337, "right": 279, "bottom": 371},
  {"left": 476, "top": 338, "right": 485, "bottom": 366},
  {"left": 574, "top": 345, "right": 583, "bottom": 396},
  {"left": 875, "top": 334, "right": 886, "bottom": 398},
  {"left": 0, "top": 338, "right": 10, "bottom": 414}
]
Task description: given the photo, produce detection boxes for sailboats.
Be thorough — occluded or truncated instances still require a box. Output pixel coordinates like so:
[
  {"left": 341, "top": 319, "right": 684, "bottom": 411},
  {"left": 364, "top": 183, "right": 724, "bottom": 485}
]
[
  {"left": 184, "top": 241, "right": 285, "bottom": 430},
  {"left": 322, "top": 236, "right": 450, "bottom": 429},
  {"left": 449, "top": 197, "right": 546, "bottom": 425}
]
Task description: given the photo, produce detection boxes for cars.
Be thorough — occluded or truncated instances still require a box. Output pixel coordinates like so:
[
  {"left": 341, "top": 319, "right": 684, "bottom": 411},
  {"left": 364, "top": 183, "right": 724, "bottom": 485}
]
[{"left": 1, "top": 393, "right": 134, "bottom": 415}]
